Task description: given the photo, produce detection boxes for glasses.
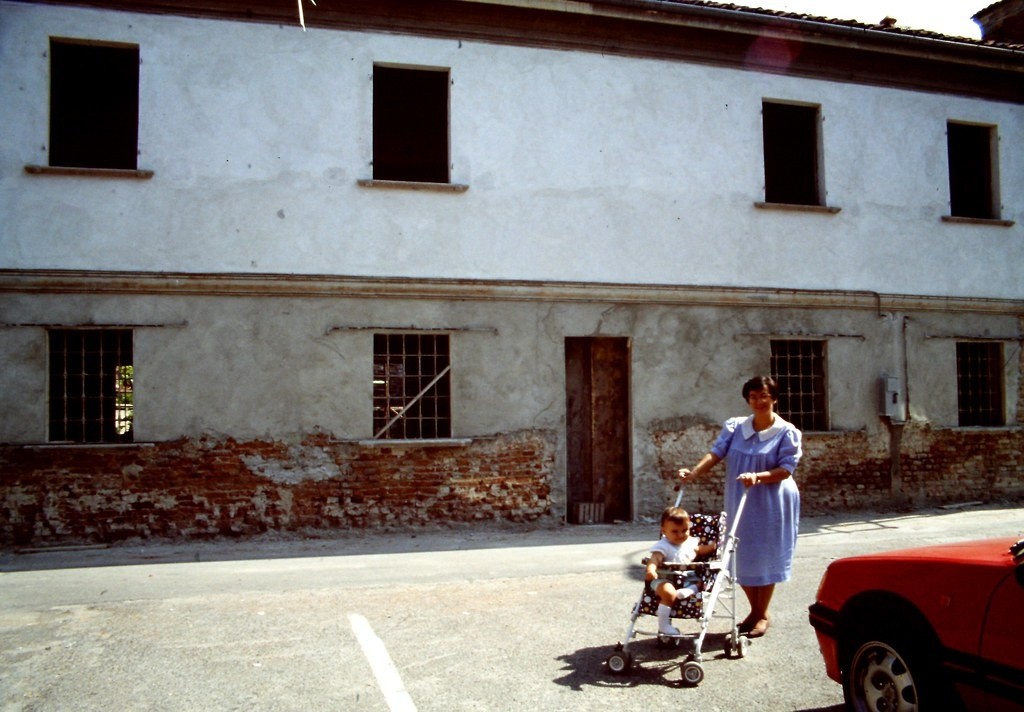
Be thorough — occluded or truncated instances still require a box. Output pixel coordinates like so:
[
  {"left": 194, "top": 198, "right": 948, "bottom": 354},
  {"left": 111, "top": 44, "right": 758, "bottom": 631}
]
[{"left": 749, "top": 392, "right": 772, "bottom": 403}]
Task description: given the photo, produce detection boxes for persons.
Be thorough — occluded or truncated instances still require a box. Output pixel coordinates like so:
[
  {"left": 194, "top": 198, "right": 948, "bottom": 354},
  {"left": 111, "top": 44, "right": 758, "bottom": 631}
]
[
  {"left": 676, "top": 375, "right": 804, "bottom": 639},
  {"left": 645, "top": 505, "right": 718, "bottom": 636}
]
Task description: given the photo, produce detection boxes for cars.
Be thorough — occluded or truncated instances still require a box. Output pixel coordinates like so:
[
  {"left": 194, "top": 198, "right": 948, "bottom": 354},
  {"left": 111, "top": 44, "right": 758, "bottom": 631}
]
[{"left": 807, "top": 533, "right": 1024, "bottom": 712}]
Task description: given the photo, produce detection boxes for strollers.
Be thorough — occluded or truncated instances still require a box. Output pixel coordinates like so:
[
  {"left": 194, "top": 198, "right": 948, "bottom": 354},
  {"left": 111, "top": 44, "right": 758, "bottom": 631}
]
[{"left": 602, "top": 478, "right": 754, "bottom": 685}]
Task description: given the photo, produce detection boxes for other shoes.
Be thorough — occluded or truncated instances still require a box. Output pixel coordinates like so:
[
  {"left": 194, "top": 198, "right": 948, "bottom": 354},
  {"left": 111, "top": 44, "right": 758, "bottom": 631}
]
[
  {"left": 748, "top": 618, "right": 771, "bottom": 638},
  {"left": 738, "top": 613, "right": 762, "bottom": 633}
]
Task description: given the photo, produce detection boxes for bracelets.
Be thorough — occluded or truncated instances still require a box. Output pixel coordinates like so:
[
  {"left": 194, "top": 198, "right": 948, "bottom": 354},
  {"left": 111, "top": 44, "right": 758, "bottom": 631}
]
[{"left": 753, "top": 471, "right": 761, "bottom": 484}]
